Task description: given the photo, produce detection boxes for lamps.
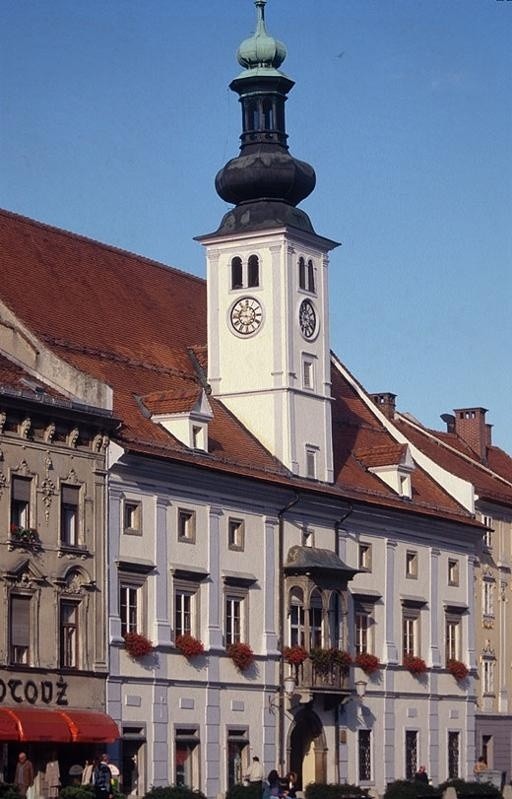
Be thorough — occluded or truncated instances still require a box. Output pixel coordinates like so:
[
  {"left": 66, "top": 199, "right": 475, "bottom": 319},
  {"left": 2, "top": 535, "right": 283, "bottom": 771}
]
[
  {"left": 341, "top": 679, "right": 368, "bottom": 715},
  {"left": 269, "top": 675, "right": 297, "bottom": 713}
]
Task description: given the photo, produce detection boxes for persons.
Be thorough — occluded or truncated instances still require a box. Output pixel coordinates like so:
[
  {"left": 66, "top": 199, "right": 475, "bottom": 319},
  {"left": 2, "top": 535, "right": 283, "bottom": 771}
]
[
  {"left": 245, "top": 756, "right": 299, "bottom": 799},
  {"left": 12, "top": 752, "right": 34, "bottom": 794},
  {"left": 472, "top": 756, "right": 488, "bottom": 782},
  {"left": 79, "top": 751, "right": 124, "bottom": 798},
  {"left": 415, "top": 764, "right": 428, "bottom": 784}
]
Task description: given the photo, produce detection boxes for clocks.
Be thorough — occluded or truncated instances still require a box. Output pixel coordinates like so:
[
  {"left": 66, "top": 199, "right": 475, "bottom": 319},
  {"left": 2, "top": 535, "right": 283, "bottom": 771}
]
[
  {"left": 225, "top": 295, "right": 263, "bottom": 338},
  {"left": 298, "top": 298, "right": 320, "bottom": 342}
]
